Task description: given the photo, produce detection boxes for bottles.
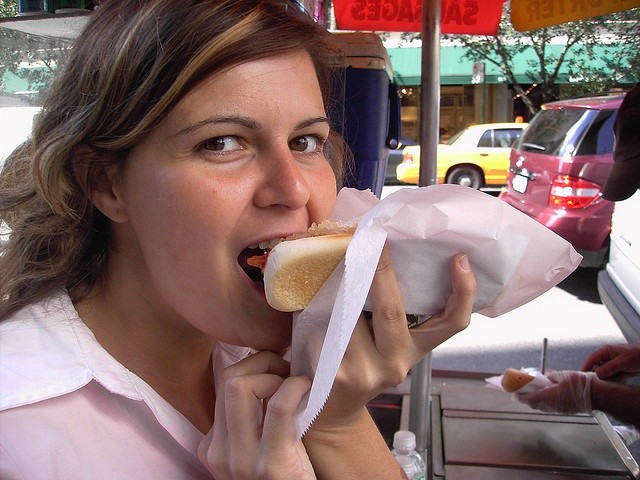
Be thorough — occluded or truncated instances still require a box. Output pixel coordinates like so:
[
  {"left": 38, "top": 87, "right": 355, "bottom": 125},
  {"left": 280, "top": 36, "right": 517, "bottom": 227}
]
[{"left": 390, "top": 430, "right": 427, "bottom": 480}]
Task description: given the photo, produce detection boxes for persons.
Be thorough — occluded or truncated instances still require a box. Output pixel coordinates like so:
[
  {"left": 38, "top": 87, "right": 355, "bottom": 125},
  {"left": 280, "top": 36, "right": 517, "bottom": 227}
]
[
  {"left": 2, "top": 0, "right": 479, "bottom": 477},
  {"left": 515, "top": 343, "right": 637, "bottom": 434}
]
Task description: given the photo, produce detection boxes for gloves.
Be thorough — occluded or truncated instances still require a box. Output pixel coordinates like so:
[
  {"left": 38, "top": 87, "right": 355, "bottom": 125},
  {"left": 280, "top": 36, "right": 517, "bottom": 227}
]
[{"left": 512, "top": 367, "right": 596, "bottom": 421}]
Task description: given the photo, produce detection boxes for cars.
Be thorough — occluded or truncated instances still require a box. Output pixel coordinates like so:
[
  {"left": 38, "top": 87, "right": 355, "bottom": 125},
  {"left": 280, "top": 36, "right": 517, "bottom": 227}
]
[
  {"left": 395, "top": 123, "right": 528, "bottom": 190},
  {"left": 385, "top": 138, "right": 414, "bottom": 184}
]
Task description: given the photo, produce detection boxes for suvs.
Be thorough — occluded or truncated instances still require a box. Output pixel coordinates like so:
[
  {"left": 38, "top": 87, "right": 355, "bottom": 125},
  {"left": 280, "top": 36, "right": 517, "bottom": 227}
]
[{"left": 498, "top": 96, "right": 627, "bottom": 267}]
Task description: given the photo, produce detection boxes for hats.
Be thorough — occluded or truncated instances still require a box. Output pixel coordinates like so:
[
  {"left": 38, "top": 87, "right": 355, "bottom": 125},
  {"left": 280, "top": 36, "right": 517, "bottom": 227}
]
[{"left": 601, "top": 82, "right": 640, "bottom": 201}]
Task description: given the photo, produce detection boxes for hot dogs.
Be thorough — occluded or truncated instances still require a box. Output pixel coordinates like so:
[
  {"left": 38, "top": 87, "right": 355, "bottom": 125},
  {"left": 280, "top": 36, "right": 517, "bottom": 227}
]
[
  {"left": 247, "top": 231, "right": 353, "bottom": 313},
  {"left": 501, "top": 368, "right": 535, "bottom": 395}
]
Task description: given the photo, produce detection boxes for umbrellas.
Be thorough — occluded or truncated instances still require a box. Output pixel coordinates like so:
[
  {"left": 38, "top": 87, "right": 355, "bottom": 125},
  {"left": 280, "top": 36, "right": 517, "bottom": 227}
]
[{"left": 299, "top": 2, "right": 640, "bottom": 475}]
